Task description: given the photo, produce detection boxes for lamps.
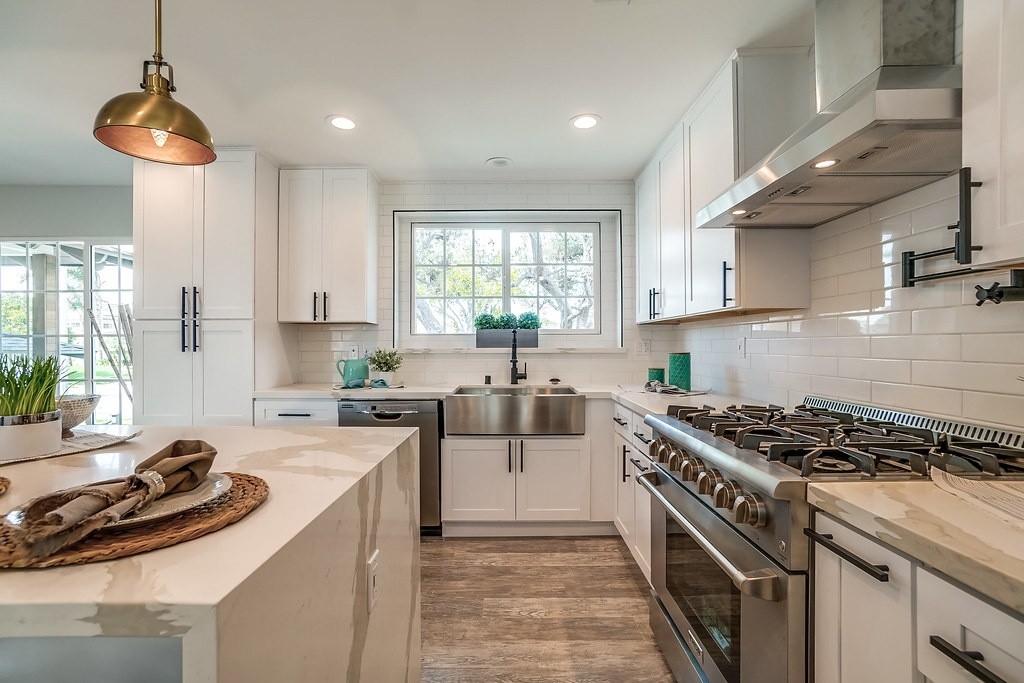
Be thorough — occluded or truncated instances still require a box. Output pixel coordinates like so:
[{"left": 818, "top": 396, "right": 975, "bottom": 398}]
[{"left": 92, "top": 0, "right": 217, "bottom": 166}]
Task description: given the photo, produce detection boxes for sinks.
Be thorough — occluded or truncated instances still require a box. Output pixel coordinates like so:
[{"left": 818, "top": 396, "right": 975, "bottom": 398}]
[{"left": 445, "top": 386, "right": 586, "bottom": 434}]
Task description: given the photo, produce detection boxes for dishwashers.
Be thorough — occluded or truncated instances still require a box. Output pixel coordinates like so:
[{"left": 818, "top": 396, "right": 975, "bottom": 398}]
[{"left": 336, "top": 400, "right": 442, "bottom": 533}]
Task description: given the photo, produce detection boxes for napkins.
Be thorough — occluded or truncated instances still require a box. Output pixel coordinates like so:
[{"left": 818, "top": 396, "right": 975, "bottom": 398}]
[{"left": 5, "top": 437, "right": 218, "bottom": 564}]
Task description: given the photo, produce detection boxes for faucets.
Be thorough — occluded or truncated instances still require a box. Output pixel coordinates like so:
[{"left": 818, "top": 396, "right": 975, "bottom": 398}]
[{"left": 510, "top": 329, "right": 527, "bottom": 385}]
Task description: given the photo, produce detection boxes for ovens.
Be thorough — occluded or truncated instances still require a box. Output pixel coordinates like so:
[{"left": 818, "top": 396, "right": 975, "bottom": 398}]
[{"left": 632, "top": 462, "right": 821, "bottom": 683}]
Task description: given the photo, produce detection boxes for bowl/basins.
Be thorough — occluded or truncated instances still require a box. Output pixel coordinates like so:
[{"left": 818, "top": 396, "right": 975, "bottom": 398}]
[{"left": 55, "top": 393, "right": 101, "bottom": 438}]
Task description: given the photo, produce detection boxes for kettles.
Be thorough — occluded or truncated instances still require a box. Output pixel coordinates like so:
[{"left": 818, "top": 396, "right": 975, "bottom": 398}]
[{"left": 336, "top": 358, "right": 369, "bottom": 386}]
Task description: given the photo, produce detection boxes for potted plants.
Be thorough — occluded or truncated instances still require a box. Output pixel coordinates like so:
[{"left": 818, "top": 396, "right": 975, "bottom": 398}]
[
  {"left": 474, "top": 314, "right": 541, "bottom": 348},
  {"left": 0, "top": 351, "right": 88, "bottom": 462},
  {"left": 365, "top": 348, "right": 403, "bottom": 385}
]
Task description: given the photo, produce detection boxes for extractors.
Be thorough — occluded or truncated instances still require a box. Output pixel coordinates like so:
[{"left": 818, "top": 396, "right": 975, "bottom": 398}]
[{"left": 696, "top": 1, "right": 962, "bottom": 233}]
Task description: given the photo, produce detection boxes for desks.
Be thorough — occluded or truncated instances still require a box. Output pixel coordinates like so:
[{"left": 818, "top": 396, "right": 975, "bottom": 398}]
[{"left": 0, "top": 425, "right": 420, "bottom": 683}]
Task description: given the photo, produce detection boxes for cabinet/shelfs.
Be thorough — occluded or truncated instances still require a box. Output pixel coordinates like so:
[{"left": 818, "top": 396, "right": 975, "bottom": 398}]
[
  {"left": 278, "top": 169, "right": 378, "bottom": 326},
  {"left": 253, "top": 398, "right": 339, "bottom": 427},
  {"left": 804, "top": 512, "right": 1024, "bottom": 683},
  {"left": 440, "top": 398, "right": 620, "bottom": 537},
  {"left": 634, "top": 53, "right": 810, "bottom": 326},
  {"left": 612, "top": 403, "right": 655, "bottom": 592},
  {"left": 959, "top": 0, "right": 1024, "bottom": 270},
  {"left": 133, "top": 150, "right": 299, "bottom": 426}
]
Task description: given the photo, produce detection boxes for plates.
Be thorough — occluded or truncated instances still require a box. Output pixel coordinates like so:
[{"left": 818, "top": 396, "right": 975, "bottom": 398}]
[{"left": 3, "top": 471, "right": 232, "bottom": 530}]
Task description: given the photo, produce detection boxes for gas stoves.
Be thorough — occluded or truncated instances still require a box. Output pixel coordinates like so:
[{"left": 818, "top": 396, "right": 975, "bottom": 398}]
[{"left": 644, "top": 391, "right": 1024, "bottom": 570}]
